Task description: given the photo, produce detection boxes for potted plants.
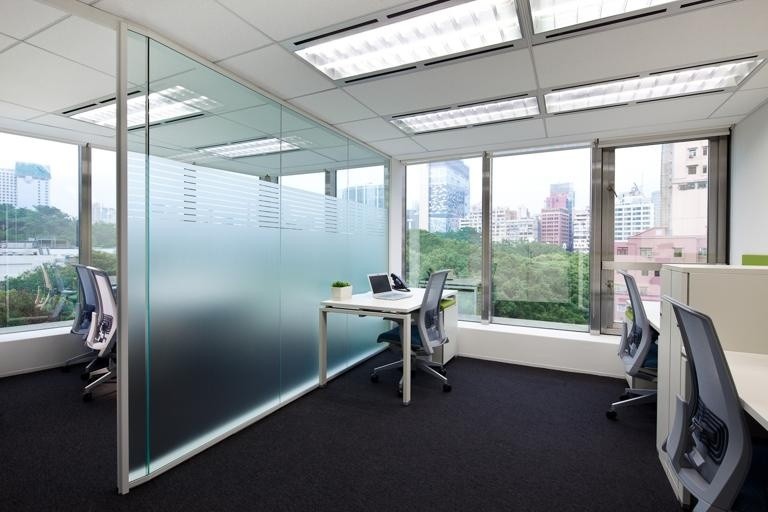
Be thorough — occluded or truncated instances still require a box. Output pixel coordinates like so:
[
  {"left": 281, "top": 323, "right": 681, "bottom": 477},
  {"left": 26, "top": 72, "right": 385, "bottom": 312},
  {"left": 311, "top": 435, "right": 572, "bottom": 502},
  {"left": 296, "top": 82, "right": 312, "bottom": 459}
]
[{"left": 331, "top": 280, "right": 352, "bottom": 302}]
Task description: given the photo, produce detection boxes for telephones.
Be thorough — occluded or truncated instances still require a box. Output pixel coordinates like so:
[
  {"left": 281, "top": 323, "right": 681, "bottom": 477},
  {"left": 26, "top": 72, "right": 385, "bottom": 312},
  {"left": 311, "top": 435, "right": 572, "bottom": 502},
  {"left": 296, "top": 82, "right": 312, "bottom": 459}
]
[{"left": 390, "top": 272, "right": 410, "bottom": 290}]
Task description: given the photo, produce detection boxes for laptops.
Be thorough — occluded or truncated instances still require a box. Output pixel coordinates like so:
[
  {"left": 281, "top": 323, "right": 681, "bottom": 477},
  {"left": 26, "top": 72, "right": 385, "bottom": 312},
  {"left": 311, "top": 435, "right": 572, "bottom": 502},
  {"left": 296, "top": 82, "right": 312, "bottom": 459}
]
[{"left": 367, "top": 273, "right": 412, "bottom": 300}]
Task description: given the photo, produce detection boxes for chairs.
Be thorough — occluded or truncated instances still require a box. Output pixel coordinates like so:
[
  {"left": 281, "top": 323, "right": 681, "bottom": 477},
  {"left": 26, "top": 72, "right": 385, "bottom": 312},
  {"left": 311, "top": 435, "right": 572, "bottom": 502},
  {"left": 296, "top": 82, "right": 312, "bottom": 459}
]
[
  {"left": 32, "top": 263, "right": 75, "bottom": 311},
  {"left": 662, "top": 294, "right": 752, "bottom": 512},
  {"left": 81, "top": 266, "right": 117, "bottom": 403},
  {"left": 64, "top": 264, "right": 104, "bottom": 380},
  {"left": 605, "top": 270, "right": 661, "bottom": 419},
  {"left": 370, "top": 269, "right": 452, "bottom": 398}
]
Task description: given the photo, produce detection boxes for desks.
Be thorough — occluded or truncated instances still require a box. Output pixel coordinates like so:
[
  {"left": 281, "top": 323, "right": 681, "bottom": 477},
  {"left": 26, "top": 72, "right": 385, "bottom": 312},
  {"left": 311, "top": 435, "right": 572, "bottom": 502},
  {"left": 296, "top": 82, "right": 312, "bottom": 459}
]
[
  {"left": 318, "top": 287, "right": 459, "bottom": 406},
  {"left": 624, "top": 298, "right": 660, "bottom": 391},
  {"left": 682, "top": 345, "right": 768, "bottom": 436}
]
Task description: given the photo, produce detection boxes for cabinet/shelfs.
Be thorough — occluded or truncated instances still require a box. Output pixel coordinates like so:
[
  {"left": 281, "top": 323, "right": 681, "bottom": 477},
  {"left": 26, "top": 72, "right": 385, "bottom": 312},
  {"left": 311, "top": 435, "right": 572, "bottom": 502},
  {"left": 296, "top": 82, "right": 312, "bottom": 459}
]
[{"left": 656, "top": 264, "right": 767, "bottom": 507}]
